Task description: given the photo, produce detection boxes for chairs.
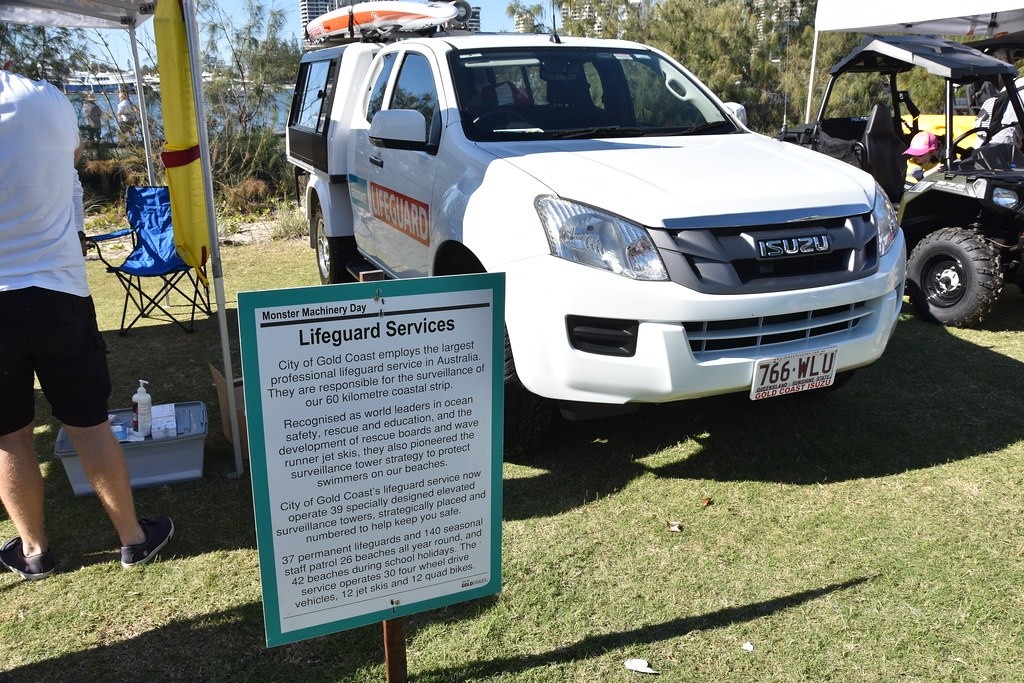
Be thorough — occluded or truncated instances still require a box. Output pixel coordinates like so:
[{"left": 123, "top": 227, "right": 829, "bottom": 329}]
[
  {"left": 546, "top": 78, "right": 587, "bottom": 111},
  {"left": 469, "top": 81, "right": 524, "bottom": 121},
  {"left": 83, "top": 185, "right": 212, "bottom": 334},
  {"left": 862, "top": 103, "right": 906, "bottom": 203}
]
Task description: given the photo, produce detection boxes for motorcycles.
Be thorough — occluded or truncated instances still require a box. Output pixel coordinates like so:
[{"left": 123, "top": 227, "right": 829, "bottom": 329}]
[{"left": 775, "top": 33, "right": 1024, "bottom": 326}]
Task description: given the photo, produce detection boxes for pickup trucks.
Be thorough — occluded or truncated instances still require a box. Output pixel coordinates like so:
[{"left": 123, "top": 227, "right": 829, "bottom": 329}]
[{"left": 283, "top": 30, "right": 907, "bottom": 457}]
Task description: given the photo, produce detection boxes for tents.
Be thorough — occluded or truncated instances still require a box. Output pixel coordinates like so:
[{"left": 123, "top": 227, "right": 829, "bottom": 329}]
[
  {"left": 804, "top": 0, "right": 1024, "bottom": 124},
  {"left": 0, "top": 0, "right": 246, "bottom": 479}
]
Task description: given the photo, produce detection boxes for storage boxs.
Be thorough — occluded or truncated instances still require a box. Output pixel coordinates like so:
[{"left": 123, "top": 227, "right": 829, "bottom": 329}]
[
  {"left": 208, "top": 362, "right": 249, "bottom": 463},
  {"left": 53, "top": 400, "right": 208, "bottom": 496}
]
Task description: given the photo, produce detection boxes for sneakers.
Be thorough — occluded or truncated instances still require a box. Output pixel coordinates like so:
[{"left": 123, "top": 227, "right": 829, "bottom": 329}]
[
  {"left": 0, "top": 536, "right": 54, "bottom": 580},
  {"left": 121, "top": 516, "right": 174, "bottom": 568}
]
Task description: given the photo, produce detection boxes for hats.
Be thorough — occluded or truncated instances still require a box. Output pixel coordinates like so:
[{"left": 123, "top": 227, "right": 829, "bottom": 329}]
[
  {"left": 83, "top": 92, "right": 97, "bottom": 101},
  {"left": 902, "top": 132, "right": 938, "bottom": 156}
]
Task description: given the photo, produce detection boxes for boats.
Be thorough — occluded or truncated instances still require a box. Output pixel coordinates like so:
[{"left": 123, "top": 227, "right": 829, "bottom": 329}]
[
  {"left": 61, "top": 70, "right": 138, "bottom": 94},
  {"left": 304, "top": 0, "right": 459, "bottom": 41},
  {"left": 145, "top": 70, "right": 217, "bottom": 91}
]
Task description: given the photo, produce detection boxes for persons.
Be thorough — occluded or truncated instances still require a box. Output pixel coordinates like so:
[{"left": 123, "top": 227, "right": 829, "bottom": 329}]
[
  {"left": 0, "top": 60, "right": 175, "bottom": 582},
  {"left": 116, "top": 88, "right": 138, "bottom": 140},
  {"left": 71, "top": 165, "right": 88, "bottom": 258},
  {"left": 902, "top": 132, "right": 946, "bottom": 191},
  {"left": 80, "top": 91, "right": 105, "bottom": 143}
]
